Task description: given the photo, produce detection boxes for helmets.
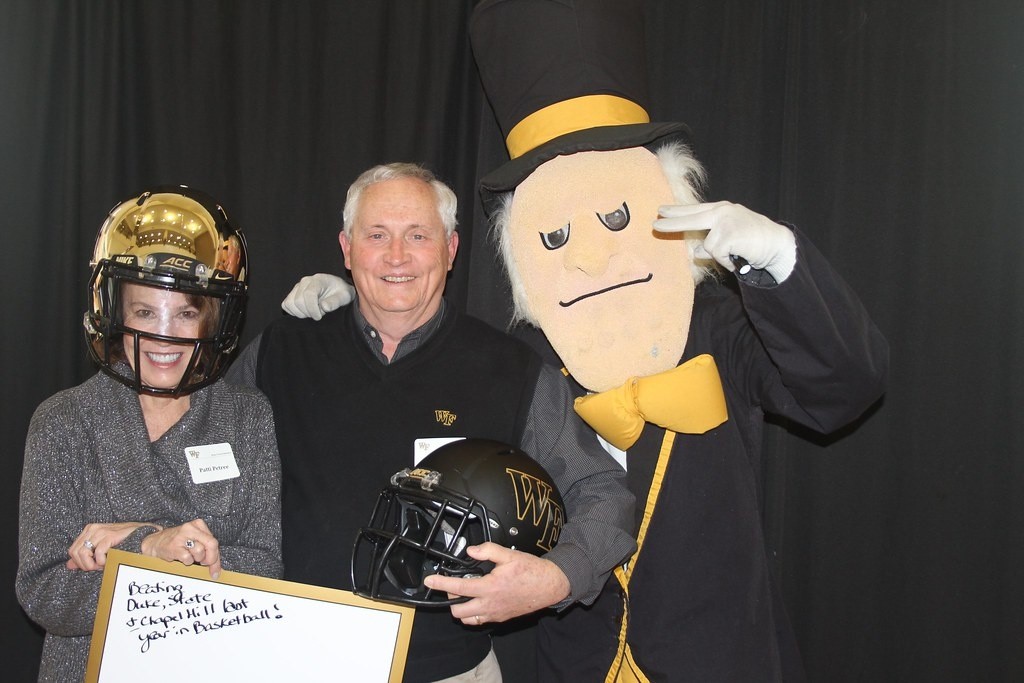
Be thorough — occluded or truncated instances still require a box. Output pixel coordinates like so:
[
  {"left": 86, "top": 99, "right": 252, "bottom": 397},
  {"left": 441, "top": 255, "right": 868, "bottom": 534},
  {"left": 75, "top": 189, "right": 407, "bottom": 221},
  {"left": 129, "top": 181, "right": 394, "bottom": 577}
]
[
  {"left": 84, "top": 184, "right": 250, "bottom": 397},
  {"left": 350, "top": 437, "right": 565, "bottom": 606}
]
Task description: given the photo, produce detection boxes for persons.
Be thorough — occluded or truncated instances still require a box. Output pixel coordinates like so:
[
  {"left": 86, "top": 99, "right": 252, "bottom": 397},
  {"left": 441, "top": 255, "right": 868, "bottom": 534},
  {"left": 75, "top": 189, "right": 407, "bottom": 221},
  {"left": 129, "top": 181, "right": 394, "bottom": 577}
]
[
  {"left": 15, "top": 185, "right": 281, "bottom": 683},
  {"left": 226, "top": 162, "right": 635, "bottom": 683},
  {"left": 285, "top": 95, "right": 889, "bottom": 683}
]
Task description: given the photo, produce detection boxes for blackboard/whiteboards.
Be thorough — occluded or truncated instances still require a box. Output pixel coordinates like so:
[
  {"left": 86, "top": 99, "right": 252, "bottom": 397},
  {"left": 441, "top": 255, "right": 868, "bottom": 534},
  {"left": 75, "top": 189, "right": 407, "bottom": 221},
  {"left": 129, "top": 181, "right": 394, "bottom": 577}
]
[{"left": 84, "top": 548, "right": 416, "bottom": 683}]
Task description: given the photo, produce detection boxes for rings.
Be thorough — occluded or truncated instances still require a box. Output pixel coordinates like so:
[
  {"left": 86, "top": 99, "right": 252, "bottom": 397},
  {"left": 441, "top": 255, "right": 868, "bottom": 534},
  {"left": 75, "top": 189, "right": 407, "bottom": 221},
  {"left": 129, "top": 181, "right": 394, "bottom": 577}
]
[
  {"left": 476, "top": 616, "right": 482, "bottom": 625},
  {"left": 185, "top": 539, "right": 194, "bottom": 549},
  {"left": 84, "top": 541, "right": 96, "bottom": 552}
]
